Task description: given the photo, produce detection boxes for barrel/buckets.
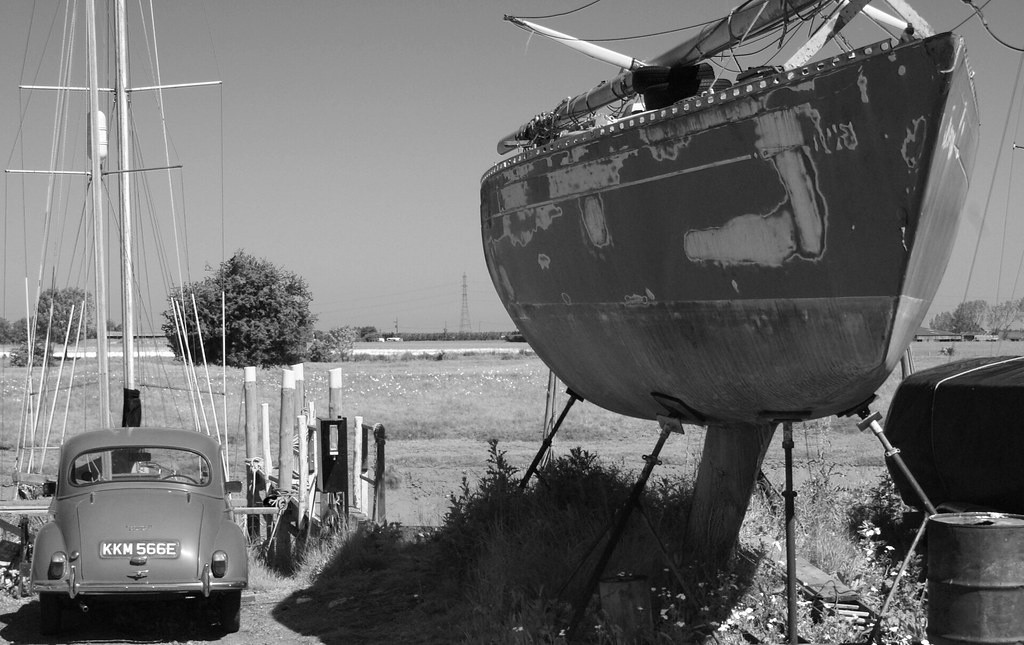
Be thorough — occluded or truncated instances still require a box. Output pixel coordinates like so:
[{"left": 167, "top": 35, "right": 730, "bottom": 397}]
[{"left": 926, "top": 512, "right": 1024, "bottom": 645}]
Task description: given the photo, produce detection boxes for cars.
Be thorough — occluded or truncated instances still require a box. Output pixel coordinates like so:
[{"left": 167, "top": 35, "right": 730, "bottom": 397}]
[{"left": 31, "top": 429, "right": 248, "bottom": 633}]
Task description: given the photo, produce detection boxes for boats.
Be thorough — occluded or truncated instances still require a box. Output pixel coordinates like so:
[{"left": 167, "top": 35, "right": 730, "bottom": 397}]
[
  {"left": 503, "top": 330, "right": 526, "bottom": 342},
  {"left": 479, "top": 0, "right": 1024, "bottom": 425}
]
